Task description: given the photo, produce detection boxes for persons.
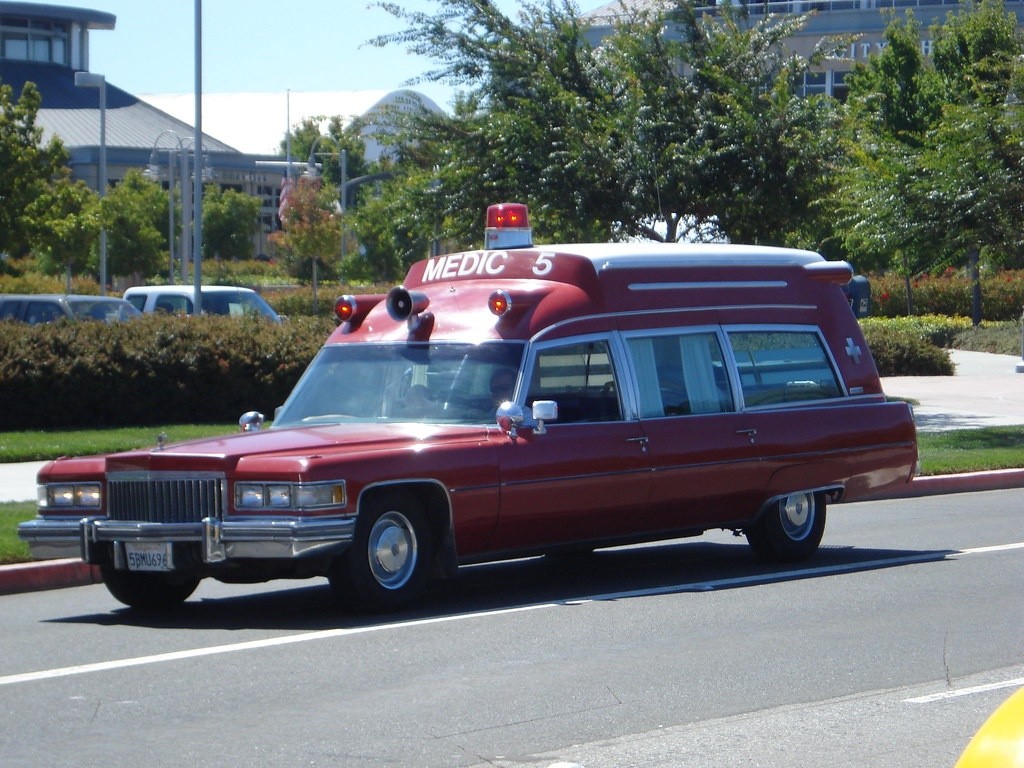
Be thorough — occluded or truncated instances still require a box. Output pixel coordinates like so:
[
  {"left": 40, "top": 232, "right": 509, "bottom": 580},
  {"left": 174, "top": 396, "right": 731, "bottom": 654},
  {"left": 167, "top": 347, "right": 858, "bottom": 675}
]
[
  {"left": 489, "top": 369, "right": 537, "bottom": 428},
  {"left": 394, "top": 385, "right": 438, "bottom": 418},
  {"left": 841, "top": 265, "right": 872, "bottom": 320}
]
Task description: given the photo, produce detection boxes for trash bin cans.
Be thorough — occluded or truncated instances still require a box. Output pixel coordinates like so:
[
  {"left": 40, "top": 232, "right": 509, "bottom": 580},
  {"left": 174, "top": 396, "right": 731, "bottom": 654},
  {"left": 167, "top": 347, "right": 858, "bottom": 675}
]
[{"left": 845, "top": 276, "right": 870, "bottom": 320}]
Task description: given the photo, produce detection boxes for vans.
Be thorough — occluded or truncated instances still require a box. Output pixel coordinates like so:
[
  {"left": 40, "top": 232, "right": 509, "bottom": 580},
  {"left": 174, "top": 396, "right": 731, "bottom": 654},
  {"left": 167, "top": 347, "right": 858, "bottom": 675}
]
[{"left": 116, "top": 284, "right": 282, "bottom": 324}]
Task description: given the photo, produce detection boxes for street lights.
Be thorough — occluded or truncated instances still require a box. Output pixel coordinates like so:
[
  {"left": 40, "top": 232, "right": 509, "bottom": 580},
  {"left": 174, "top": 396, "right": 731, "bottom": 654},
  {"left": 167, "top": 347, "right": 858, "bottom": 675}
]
[
  {"left": 74, "top": 70, "right": 109, "bottom": 294},
  {"left": 149, "top": 130, "right": 214, "bottom": 281},
  {"left": 307, "top": 134, "right": 349, "bottom": 261}
]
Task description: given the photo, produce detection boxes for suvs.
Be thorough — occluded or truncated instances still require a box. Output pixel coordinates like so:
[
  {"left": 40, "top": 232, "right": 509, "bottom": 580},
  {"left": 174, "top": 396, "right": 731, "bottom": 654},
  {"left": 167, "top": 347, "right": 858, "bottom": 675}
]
[
  {"left": 1, "top": 292, "right": 143, "bottom": 326},
  {"left": 14, "top": 202, "right": 919, "bottom": 617}
]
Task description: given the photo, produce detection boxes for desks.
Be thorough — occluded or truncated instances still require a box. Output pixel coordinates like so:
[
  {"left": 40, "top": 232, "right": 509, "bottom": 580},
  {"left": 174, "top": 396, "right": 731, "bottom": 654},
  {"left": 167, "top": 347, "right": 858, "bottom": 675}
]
[{"left": 527, "top": 393, "right": 621, "bottom": 425}]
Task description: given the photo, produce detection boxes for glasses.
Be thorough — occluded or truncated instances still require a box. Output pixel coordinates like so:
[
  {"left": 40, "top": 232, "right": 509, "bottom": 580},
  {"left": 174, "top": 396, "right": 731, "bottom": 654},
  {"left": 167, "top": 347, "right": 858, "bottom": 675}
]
[{"left": 489, "top": 382, "right": 515, "bottom": 393}]
